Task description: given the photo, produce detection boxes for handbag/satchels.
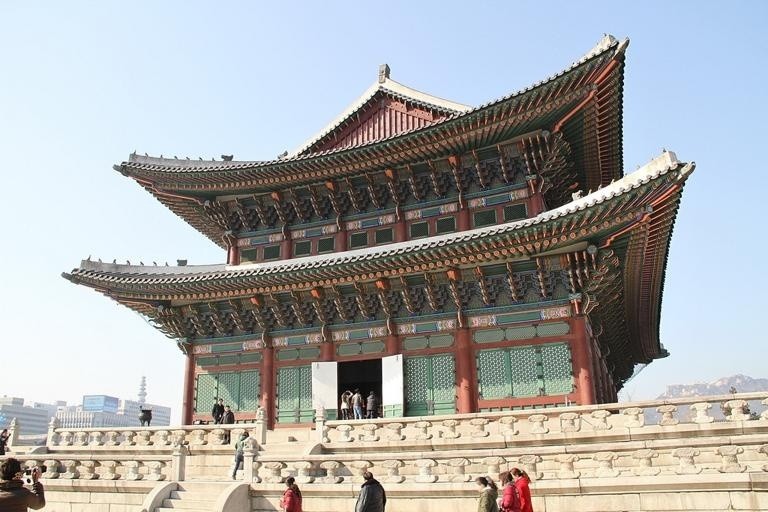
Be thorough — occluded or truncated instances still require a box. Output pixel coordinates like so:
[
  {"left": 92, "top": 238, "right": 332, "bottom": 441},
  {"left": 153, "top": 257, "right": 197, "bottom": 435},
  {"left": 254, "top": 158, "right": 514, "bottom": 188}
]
[{"left": 499, "top": 503, "right": 509, "bottom": 512}]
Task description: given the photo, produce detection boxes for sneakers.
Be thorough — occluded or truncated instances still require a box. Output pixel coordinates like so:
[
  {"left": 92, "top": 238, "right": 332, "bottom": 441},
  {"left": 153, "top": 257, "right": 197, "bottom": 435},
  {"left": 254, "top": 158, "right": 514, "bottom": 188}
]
[{"left": 231, "top": 475, "right": 236, "bottom": 480}]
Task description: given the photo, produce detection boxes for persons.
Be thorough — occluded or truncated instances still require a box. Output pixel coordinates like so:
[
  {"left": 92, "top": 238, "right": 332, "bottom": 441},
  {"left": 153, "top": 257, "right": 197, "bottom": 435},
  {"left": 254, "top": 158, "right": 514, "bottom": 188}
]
[
  {"left": 486, "top": 476, "right": 497, "bottom": 490},
  {"left": 355, "top": 471, "right": 386, "bottom": 512},
  {"left": 363, "top": 391, "right": 377, "bottom": 418},
  {"left": 232, "top": 432, "right": 249, "bottom": 480},
  {"left": 351, "top": 388, "right": 363, "bottom": 419},
  {"left": 341, "top": 390, "right": 354, "bottom": 420},
  {"left": 498, "top": 472, "right": 521, "bottom": 512},
  {"left": 279, "top": 477, "right": 302, "bottom": 512},
  {"left": 0, "top": 429, "right": 11, "bottom": 455},
  {"left": 212, "top": 397, "right": 234, "bottom": 444},
  {"left": 0, "top": 458, "right": 45, "bottom": 512},
  {"left": 510, "top": 468, "right": 533, "bottom": 512},
  {"left": 475, "top": 477, "right": 498, "bottom": 512}
]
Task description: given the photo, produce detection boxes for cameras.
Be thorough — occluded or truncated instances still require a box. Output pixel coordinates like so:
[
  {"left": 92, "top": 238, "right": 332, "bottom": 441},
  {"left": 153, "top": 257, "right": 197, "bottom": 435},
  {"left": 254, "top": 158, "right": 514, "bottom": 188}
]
[{"left": 26, "top": 467, "right": 36, "bottom": 475}]
